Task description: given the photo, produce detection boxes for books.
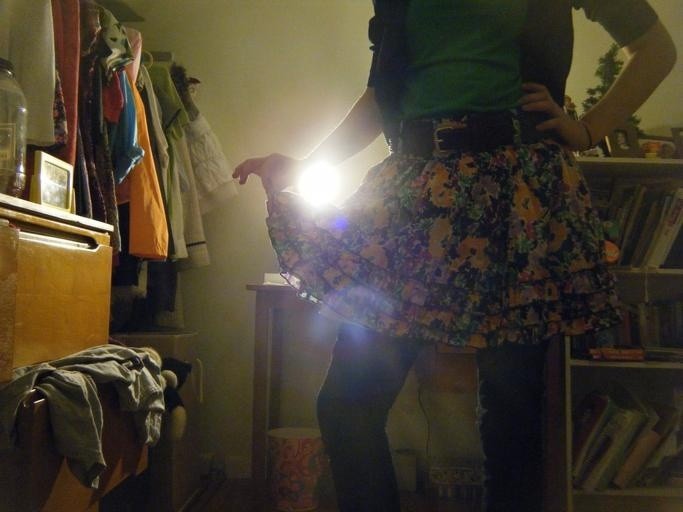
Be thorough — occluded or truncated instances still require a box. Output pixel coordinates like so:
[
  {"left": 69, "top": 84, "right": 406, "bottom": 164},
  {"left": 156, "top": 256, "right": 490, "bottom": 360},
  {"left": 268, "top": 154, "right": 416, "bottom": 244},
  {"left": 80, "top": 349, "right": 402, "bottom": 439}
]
[
  {"left": 591, "top": 181, "right": 683, "bottom": 271},
  {"left": 574, "top": 384, "right": 682, "bottom": 492},
  {"left": 586, "top": 300, "right": 682, "bottom": 365}
]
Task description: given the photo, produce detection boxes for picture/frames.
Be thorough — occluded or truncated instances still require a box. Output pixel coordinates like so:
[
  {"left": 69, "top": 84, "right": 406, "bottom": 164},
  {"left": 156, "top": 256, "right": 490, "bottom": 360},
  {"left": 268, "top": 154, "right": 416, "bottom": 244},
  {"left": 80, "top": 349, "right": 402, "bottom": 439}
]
[{"left": 34, "top": 150, "right": 73, "bottom": 211}]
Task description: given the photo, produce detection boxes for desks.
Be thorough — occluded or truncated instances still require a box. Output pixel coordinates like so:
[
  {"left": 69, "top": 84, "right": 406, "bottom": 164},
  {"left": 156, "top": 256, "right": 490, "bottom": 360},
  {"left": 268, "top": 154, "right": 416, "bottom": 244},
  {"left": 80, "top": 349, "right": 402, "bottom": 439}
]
[{"left": 244, "top": 283, "right": 329, "bottom": 491}]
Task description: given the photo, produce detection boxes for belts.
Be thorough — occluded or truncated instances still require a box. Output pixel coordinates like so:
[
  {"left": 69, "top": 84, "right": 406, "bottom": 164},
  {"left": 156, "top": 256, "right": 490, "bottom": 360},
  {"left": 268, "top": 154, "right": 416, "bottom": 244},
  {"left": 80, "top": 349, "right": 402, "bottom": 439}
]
[{"left": 382, "top": 111, "right": 559, "bottom": 160}]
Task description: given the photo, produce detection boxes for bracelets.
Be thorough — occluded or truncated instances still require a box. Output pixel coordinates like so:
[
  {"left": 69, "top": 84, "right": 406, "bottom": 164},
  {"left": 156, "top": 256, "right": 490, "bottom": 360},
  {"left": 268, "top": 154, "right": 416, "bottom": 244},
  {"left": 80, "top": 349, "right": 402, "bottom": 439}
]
[{"left": 581, "top": 122, "right": 593, "bottom": 152}]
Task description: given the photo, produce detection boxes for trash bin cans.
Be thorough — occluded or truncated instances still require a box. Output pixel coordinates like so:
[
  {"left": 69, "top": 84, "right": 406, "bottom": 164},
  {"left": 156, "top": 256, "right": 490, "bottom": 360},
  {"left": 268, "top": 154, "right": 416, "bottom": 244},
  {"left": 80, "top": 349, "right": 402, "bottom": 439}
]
[{"left": 267, "top": 428, "right": 327, "bottom": 512}]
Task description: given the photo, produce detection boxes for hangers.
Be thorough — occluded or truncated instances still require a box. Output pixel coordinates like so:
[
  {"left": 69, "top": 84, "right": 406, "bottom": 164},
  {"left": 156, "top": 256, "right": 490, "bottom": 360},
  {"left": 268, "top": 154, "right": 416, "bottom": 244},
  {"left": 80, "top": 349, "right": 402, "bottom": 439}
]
[{"left": 133, "top": 39, "right": 201, "bottom": 93}]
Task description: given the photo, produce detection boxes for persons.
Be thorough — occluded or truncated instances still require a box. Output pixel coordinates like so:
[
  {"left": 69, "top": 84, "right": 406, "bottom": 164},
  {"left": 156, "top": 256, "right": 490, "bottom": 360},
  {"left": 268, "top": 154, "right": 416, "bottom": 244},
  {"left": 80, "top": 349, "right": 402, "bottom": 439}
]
[{"left": 231, "top": 0, "right": 677, "bottom": 512}]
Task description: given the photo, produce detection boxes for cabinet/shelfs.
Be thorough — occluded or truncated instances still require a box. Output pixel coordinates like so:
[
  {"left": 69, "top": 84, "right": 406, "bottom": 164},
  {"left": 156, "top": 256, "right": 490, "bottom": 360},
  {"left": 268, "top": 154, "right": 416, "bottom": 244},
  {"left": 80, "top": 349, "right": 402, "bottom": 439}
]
[
  {"left": 0, "top": 194, "right": 114, "bottom": 511},
  {"left": 564, "top": 155, "right": 683, "bottom": 512}
]
